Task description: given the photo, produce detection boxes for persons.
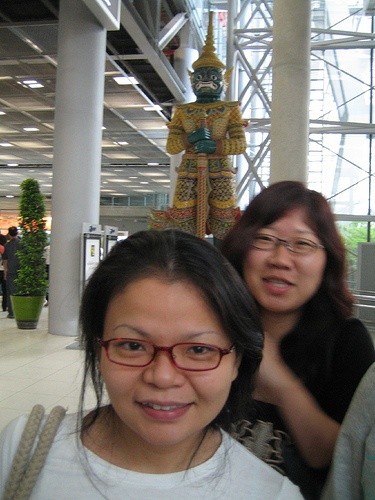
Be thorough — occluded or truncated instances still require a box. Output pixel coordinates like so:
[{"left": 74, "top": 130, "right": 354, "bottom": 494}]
[
  {"left": 0, "top": 229, "right": 305, "bottom": 500},
  {"left": 213, "top": 181, "right": 375, "bottom": 500},
  {"left": 0, "top": 223, "right": 51, "bottom": 318},
  {"left": 146, "top": 11, "right": 248, "bottom": 241}
]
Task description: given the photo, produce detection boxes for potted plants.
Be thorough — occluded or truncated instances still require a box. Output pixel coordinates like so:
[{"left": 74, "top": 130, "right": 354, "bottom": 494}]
[{"left": 10, "top": 178, "right": 50, "bottom": 331}]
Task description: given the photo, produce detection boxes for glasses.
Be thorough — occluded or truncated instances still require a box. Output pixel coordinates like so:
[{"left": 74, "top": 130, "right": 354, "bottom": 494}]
[
  {"left": 93, "top": 335, "right": 235, "bottom": 371},
  {"left": 249, "top": 232, "right": 326, "bottom": 256}
]
